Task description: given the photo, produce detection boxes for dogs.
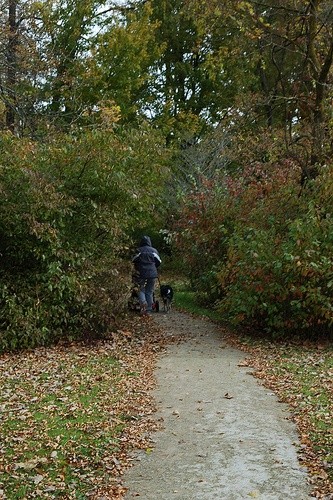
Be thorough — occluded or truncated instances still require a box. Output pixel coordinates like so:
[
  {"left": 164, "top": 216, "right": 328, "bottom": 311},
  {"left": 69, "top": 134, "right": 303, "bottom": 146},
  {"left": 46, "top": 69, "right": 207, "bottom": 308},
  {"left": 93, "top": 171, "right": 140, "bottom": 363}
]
[{"left": 160, "top": 284, "right": 174, "bottom": 313}]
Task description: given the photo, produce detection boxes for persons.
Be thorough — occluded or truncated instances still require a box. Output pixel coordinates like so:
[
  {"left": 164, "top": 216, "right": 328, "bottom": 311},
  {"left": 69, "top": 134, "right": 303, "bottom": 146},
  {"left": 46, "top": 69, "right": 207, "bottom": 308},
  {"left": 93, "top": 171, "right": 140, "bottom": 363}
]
[{"left": 131, "top": 236, "right": 162, "bottom": 316}]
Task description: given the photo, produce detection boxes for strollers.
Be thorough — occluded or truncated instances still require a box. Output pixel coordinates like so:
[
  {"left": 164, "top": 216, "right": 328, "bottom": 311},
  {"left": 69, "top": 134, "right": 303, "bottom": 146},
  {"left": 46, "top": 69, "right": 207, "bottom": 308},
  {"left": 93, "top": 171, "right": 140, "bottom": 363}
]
[{"left": 128, "top": 276, "right": 160, "bottom": 312}]
